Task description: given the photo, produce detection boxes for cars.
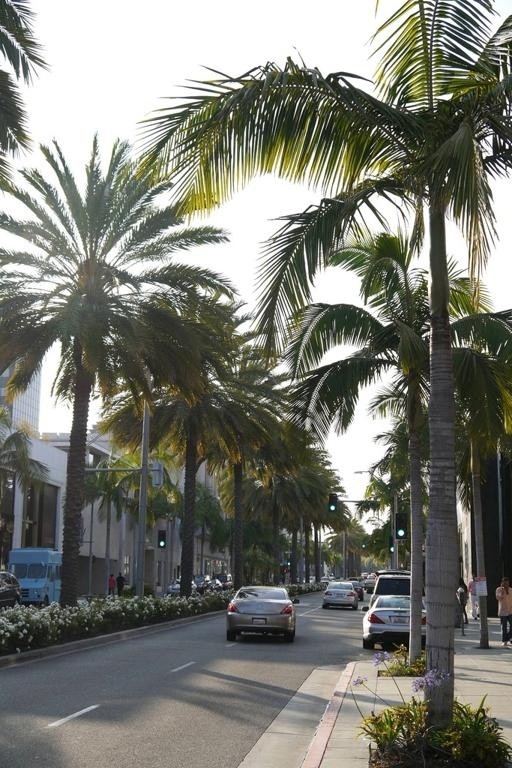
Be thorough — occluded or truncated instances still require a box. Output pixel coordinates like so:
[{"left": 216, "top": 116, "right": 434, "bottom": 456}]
[
  {"left": 322, "top": 569, "right": 426, "bottom": 650},
  {"left": 226, "top": 586, "right": 300, "bottom": 640},
  {"left": 167, "top": 573, "right": 234, "bottom": 597},
  {"left": 0, "top": 571, "right": 23, "bottom": 611}
]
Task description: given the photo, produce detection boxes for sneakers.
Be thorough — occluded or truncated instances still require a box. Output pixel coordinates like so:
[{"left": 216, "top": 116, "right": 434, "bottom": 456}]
[{"left": 475, "top": 614, "right": 480, "bottom": 620}]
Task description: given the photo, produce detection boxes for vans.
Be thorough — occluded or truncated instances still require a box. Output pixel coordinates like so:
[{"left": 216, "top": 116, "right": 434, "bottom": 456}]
[{"left": 7, "top": 545, "right": 63, "bottom": 608}]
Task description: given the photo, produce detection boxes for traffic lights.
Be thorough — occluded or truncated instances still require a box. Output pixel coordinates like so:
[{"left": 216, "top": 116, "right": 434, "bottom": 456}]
[
  {"left": 395, "top": 512, "right": 408, "bottom": 539},
  {"left": 157, "top": 529, "right": 167, "bottom": 548},
  {"left": 328, "top": 493, "right": 338, "bottom": 512}
]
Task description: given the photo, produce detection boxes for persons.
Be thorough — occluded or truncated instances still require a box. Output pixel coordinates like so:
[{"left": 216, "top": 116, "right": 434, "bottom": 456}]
[
  {"left": 108, "top": 574, "right": 116, "bottom": 595},
  {"left": 495, "top": 576, "right": 512, "bottom": 646},
  {"left": 460, "top": 577, "right": 469, "bottom": 624},
  {"left": 116, "top": 572, "right": 124, "bottom": 597},
  {"left": 467, "top": 574, "right": 480, "bottom": 620},
  {"left": 296, "top": 576, "right": 306, "bottom": 584}
]
